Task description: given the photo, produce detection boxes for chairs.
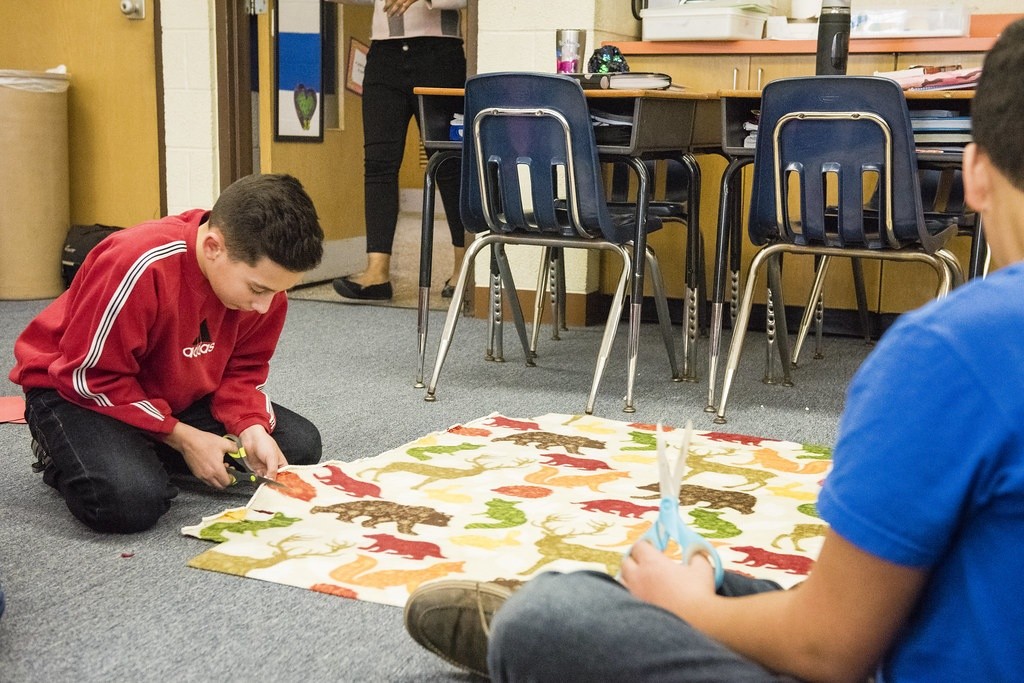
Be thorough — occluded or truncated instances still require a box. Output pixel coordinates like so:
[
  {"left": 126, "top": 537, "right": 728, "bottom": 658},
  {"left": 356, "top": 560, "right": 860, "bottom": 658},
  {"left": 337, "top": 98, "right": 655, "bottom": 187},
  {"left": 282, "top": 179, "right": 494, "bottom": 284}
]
[{"left": 420, "top": 69, "right": 968, "bottom": 425}]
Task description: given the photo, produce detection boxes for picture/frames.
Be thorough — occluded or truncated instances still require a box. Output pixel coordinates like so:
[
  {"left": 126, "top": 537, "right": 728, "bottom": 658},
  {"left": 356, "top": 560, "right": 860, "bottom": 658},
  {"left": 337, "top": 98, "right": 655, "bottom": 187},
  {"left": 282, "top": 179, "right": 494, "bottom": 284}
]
[{"left": 273, "top": 0, "right": 327, "bottom": 143}]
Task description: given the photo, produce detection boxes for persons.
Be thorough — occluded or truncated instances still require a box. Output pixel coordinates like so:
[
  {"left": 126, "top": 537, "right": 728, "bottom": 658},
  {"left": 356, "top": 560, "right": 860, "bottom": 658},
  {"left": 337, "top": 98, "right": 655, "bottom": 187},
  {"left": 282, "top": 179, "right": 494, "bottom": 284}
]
[
  {"left": 404, "top": 17, "right": 1024, "bottom": 683},
  {"left": 8, "top": 172, "right": 323, "bottom": 536},
  {"left": 332, "top": 0, "right": 471, "bottom": 301}
]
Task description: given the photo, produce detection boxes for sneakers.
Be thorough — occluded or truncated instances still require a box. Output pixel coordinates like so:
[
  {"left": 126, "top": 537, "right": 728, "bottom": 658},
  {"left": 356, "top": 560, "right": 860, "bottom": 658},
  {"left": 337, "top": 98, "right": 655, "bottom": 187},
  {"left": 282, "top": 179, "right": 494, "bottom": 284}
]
[{"left": 404, "top": 579, "right": 515, "bottom": 683}]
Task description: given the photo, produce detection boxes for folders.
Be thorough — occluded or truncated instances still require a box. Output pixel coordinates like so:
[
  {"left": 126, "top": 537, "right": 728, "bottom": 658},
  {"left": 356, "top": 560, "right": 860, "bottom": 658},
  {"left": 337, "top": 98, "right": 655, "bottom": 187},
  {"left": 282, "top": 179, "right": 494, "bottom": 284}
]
[{"left": 559, "top": 71, "right": 672, "bottom": 91}]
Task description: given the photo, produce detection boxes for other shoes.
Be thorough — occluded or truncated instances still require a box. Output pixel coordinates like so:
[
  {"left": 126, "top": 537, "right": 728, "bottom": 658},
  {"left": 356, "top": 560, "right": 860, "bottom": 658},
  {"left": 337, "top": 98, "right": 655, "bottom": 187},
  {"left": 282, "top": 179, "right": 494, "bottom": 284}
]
[
  {"left": 442, "top": 275, "right": 466, "bottom": 297},
  {"left": 335, "top": 279, "right": 395, "bottom": 302}
]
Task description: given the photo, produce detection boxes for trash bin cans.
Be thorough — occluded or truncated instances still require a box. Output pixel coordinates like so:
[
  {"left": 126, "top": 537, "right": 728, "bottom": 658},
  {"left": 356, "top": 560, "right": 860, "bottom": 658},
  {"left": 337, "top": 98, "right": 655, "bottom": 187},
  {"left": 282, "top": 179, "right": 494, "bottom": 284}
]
[{"left": 0, "top": 69, "right": 72, "bottom": 300}]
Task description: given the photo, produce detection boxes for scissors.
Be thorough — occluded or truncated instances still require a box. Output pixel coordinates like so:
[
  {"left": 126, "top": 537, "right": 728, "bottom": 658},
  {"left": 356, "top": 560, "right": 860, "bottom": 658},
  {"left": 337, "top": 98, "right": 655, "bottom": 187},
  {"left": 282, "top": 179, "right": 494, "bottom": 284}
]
[
  {"left": 221, "top": 433, "right": 287, "bottom": 488},
  {"left": 616, "top": 419, "right": 724, "bottom": 597}
]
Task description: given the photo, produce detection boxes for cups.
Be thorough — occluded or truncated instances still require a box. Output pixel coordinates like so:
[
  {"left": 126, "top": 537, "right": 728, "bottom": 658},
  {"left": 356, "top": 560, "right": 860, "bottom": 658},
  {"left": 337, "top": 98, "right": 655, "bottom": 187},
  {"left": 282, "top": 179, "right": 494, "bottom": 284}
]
[{"left": 556, "top": 29, "right": 586, "bottom": 73}]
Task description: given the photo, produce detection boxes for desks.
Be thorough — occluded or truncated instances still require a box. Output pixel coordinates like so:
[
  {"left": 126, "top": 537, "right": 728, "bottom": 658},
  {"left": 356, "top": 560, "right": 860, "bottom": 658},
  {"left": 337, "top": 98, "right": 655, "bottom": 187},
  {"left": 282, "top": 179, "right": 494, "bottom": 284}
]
[{"left": 408, "top": 80, "right": 980, "bottom": 394}]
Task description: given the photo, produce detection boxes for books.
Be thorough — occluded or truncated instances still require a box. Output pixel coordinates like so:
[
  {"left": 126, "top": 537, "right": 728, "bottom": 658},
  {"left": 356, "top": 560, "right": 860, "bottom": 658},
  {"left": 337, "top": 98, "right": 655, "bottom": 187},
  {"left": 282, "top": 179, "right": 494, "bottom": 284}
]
[
  {"left": 910, "top": 116, "right": 976, "bottom": 131},
  {"left": 742, "top": 108, "right": 762, "bottom": 149},
  {"left": 910, "top": 143, "right": 971, "bottom": 154},
  {"left": 874, "top": 62, "right": 964, "bottom": 80},
  {"left": 910, "top": 132, "right": 978, "bottom": 146},
  {"left": 452, "top": 112, "right": 465, "bottom": 120},
  {"left": 675, "top": 0, "right": 780, "bottom": 15},
  {"left": 555, "top": 73, "right": 672, "bottom": 92},
  {"left": 588, "top": 103, "right": 634, "bottom": 131},
  {"left": 449, "top": 118, "right": 464, "bottom": 127},
  {"left": 907, "top": 108, "right": 964, "bottom": 120},
  {"left": 663, "top": 83, "right": 690, "bottom": 91}
]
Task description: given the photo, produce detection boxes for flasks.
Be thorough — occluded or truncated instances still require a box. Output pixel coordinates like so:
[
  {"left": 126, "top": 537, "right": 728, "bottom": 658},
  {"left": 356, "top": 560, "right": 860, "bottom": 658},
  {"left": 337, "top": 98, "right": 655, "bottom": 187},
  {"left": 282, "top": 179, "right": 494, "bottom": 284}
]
[{"left": 815, "top": 0, "right": 851, "bottom": 75}]
